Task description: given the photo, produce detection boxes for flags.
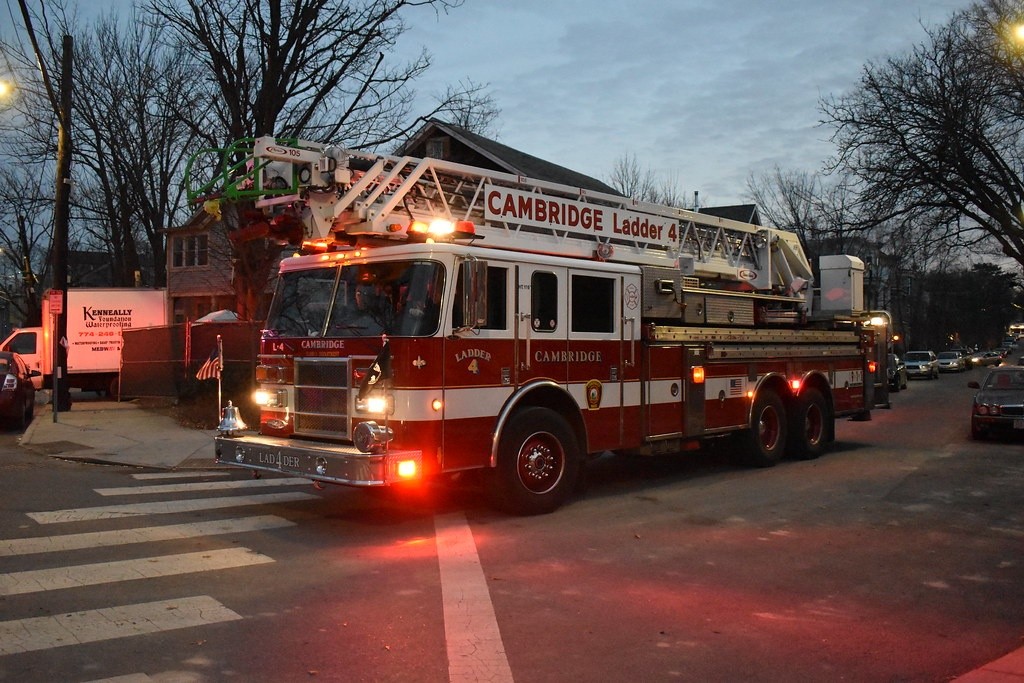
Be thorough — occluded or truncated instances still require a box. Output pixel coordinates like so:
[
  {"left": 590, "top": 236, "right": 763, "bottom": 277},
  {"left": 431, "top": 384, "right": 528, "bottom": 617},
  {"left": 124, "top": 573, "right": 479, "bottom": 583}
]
[
  {"left": 196, "top": 347, "right": 220, "bottom": 380},
  {"left": 358, "top": 342, "right": 393, "bottom": 401}
]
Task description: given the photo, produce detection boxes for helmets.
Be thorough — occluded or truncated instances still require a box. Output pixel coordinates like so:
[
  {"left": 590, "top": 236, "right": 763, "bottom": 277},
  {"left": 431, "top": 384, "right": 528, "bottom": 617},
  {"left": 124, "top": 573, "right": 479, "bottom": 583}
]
[{"left": 353, "top": 266, "right": 377, "bottom": 284}]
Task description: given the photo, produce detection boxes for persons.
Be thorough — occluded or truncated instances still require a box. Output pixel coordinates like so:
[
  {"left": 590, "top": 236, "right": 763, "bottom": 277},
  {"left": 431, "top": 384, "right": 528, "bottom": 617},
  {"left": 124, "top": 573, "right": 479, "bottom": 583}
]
[{"left": 329, "top": 280, "right": 439, "bottom": 337}]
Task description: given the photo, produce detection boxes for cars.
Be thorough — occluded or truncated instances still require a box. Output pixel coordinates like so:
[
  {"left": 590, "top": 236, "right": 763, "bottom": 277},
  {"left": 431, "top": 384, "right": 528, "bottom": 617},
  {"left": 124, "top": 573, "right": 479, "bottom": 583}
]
[
  {"left": 968, "top": 332, "right": 1020, "bottom": 366},
  {"left": 967, "top": 366, "right": 1024, "bottom": 442},
  {"left": 0, "top": 352, "right": 41, "bottom": 432},
  {"left": 937, "top": 351, "right": 965, "bottom": 373},
  {"left": 887, "top": 353, "right": 907, "bottom": 392},
  {"left": 901, "top": 351, "right": 939, "bottom": 380}
]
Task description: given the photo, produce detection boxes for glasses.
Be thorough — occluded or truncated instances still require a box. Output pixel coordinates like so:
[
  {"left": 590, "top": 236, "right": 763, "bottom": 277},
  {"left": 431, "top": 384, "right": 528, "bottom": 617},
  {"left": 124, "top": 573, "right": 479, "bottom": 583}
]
[{"left": 356, "top": 290, "right": 368, "bottom": 295}]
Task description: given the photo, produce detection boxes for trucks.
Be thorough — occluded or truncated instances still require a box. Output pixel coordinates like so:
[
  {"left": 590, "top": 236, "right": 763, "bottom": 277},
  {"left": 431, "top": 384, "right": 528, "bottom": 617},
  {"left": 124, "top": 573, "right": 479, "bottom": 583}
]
[{"left": 0, "top": 288, "right": 168, "bottom": 401}]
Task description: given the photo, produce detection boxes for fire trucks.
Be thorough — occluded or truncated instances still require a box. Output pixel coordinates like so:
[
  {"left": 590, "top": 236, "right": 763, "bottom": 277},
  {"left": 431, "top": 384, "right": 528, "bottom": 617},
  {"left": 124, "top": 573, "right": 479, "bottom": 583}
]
[{"left": 184, "top": 136, "right": 892, "bottom": 515}]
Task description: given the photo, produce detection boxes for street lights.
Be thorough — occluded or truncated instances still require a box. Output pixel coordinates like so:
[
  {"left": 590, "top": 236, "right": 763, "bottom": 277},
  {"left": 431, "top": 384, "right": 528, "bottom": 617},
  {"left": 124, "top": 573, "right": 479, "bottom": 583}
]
[{"left": 1, "top": 35, "right": 72, "bottom": 412}]
